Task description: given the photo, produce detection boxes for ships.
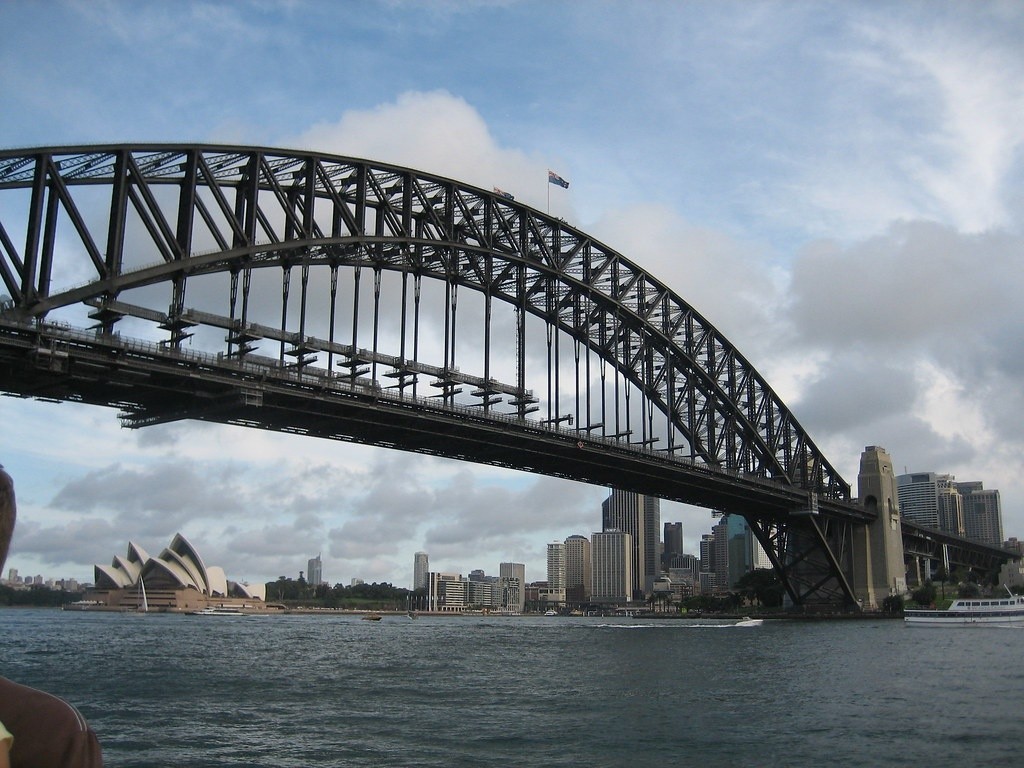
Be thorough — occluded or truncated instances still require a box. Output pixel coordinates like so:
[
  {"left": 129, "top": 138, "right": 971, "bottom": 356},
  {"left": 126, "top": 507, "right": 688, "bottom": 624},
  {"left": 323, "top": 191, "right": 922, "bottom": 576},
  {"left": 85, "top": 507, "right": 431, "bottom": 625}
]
[{"left": 902, "top": 584, "right": 1024, "bottom": 626}]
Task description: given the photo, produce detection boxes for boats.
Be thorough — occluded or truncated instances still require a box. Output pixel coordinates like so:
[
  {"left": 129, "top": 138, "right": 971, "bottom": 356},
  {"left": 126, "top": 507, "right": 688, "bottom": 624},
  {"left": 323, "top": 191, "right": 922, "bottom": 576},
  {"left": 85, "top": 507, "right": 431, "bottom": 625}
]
[
  {"left": 184, "top": 606, "right": 250, "bottom": 617},
  {"left": 735, "top": 617, "right": 764, "bottom": 627},
  {"left": 361, "top": 612, "right": 382, "bottom": 621},
  {"left": 62, "top": 601, "right": 127, "bottom": 611},
  {"left": 544, "top": 609, "right": 557, "bottom": 617}
]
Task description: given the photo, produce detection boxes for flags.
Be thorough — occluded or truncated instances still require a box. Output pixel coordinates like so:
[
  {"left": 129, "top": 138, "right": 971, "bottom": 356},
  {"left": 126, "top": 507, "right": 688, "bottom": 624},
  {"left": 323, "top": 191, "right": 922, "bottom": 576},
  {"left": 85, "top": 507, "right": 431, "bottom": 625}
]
[
  {"left": 492, "top": 187, "right": 515, "bottom": 201},
  {"left": 548, "top": 171, "right": 570, "bottom": 189}
]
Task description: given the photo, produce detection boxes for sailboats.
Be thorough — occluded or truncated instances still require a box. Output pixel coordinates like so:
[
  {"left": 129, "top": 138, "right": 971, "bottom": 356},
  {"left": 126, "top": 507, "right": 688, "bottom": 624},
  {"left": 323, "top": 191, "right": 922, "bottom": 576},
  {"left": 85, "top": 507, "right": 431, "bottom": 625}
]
[{"left": 137, "top": 574, "right": 149, "bottom": 613}]
